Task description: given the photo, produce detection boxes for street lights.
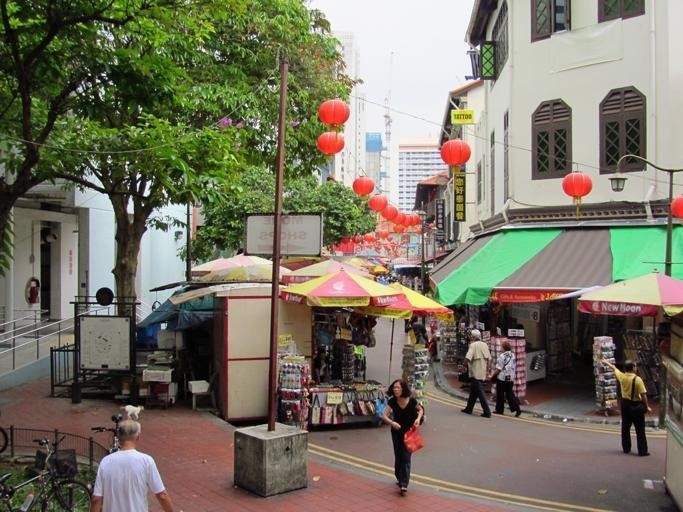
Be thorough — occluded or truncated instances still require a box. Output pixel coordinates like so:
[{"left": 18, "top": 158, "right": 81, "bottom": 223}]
[
  {"left": 416, "top": 201, "right": 435, "bottom": 295},
  {"left": 609, "top": 153, "right": 683, "bottom": 428}
]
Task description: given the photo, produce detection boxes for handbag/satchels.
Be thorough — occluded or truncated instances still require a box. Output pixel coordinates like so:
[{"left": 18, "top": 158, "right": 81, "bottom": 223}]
[
  {"left": 458, "top": 372, "right": 469, "bottom": 383},
  {"left": 407, "top": 329, "right": 417, "bottom": 345},
  {"left": 490, "top": 374, "right": 498, "bottom": 384},
  {"left": 629, "top": 402, "right": 646, "bottom": 417},
  {"left": 404, "top": 427, "right": 424, "bottom": 452}
]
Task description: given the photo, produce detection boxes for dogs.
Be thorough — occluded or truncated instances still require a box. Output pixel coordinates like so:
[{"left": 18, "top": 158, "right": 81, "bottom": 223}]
[{"left": 120, "top": 405, "right": 144, "bottom": 420}]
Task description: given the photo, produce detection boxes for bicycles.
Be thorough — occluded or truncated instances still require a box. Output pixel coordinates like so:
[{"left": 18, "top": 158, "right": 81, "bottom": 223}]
[
  {"left": 92, "top": 413, "right": 124, "bottom": 453},
  {"left": 0, "top": 426, "right": 9, "bottom": 453},
  {"left": 1, "top": 436, "right": 91, "bottom": 511}
]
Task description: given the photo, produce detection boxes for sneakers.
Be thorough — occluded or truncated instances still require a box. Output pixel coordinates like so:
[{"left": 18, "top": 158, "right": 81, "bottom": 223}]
[
  {"left": 493, "top": 411, "right": 504, "bottom": 415},
  {"left": 639, "top": 453, "right": 651, "bottom": 457},
  {"left": 461, "top": 409, "right": 472, "bottom": 414},
  {"left": 480, "top": 414, "right": 491, "bottom": 418},
  {"left": 515, "top": 410, "right": 522, "bottom": 417},
  {"left": 401, "top": 489, "right": 407, "bottom": 497},
  {"left": 396, "top": 481, "right": 401, "bottom": 488}
]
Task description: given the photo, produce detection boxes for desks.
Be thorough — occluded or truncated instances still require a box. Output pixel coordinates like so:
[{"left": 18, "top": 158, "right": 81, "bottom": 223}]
[{"left": 309, "top": 388, "right": 394, "bottom": 433}]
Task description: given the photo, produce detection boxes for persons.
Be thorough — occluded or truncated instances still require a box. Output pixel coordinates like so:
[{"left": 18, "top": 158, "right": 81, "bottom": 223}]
[
  {"left": 92, "top": 420, "right": 174, "bottom": 512},
  {"left": 413, "top": 318, "right": 426, "bottom": 345},
  {"left": 382, "top": 380, "right": 425, "bottom": 495},
  {"left": 491, "top": 341, "right": 522, "bottom": 417},
  {"left": 601, "top": 359, "right": 652, "bottom": 456},
  {"left": 461, "top": 330, "right": 492, "bottom": 416}
]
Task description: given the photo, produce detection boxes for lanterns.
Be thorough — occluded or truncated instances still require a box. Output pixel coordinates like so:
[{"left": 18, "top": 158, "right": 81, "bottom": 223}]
[
  {"left": 319, "top": 90, "right": 350, "bottom": 128},
  {"left": 332, "top": 176, "right": 423, "bottom": 257},
  {"left": 671, "top": 195, "right": 683, "bottom": 218},
  {"left": 562, "top": 164, "right": 593, "bottom": 219},
  {"left": 317, "top": 125, "right": 344, "bottom": 156},
  {"left": 440, "top": 129, "right": 471, "bottom": 177}
]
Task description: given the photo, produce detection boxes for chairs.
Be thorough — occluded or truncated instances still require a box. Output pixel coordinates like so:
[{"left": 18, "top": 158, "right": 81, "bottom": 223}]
[{"left": 192, "top": 371, "right": 218, "bottom": 413}]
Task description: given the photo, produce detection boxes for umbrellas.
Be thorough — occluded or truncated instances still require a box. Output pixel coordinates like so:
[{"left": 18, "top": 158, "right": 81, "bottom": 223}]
[
  {"left": 191, "top": 254, "right": 292, "bottom": 283},
  {"left": 368, "top": 265, "right": 387, "bottom": 274},
  {"left": 577, "top": 269, "right": 683, "bottom": 356},
  {"left": 281, "top": 267, "right": 406, "bottom": 305},
  {"left": 336, "top": 255, "right": 374, "bottom": 267},
  {"left": 289, "top": 258, "right": 377, "bottom": 279},
  {"left": 355, "top": 281, "right": 452, "bottom": 386}
]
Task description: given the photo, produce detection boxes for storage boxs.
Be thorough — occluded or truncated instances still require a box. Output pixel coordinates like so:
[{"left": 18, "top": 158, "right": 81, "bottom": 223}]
[
  {"left": 158, "top": 383, "right": 178, "bottom": 395},
  {"left": 159, "top": 396, "right": 176, "bottom": 404},
  {"left": 122, "top": 383, "right": 150, "bottom": 396},
  {"left": 188, "top": 381, "right": 209, "bottom": 393},
  {"left": 143, "top": 368, "right": 174, "bottom": 382},
  {"left": 158, "top": 330, "right": 183, "bottom": 349}
]
